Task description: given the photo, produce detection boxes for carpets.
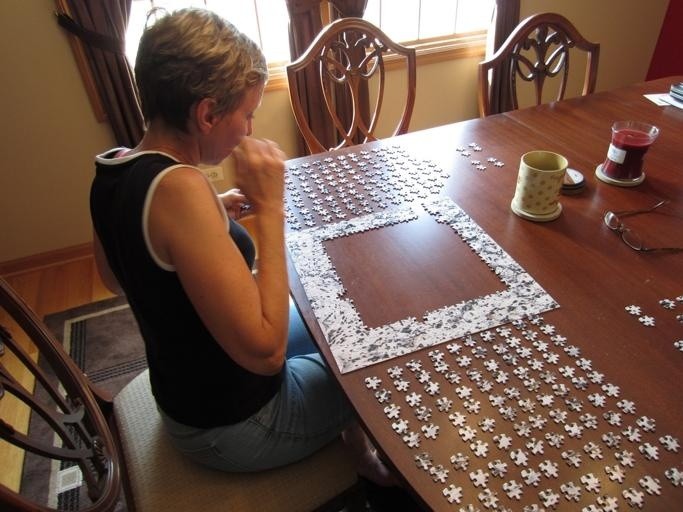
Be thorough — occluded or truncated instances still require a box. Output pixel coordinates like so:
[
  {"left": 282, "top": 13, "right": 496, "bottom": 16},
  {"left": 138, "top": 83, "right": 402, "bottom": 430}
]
[{"left": 18, "top": 257, "right": 435, "bottom": 512}]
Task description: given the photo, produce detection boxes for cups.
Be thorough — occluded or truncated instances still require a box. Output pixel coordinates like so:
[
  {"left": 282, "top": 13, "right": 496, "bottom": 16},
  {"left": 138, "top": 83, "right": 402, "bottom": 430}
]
[
  {"left": 512, "top": 149, "right": 569, "bottom": 216},
  {"left": 602, "top": 120, "right": 660, "bottom": 182}
]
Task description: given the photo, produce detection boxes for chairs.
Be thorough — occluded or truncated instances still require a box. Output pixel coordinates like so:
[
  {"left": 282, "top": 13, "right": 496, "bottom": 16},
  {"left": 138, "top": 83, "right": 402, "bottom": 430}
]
[
  {"left": 285, "top": 17, "right": 416, "bottom": 154},
  {"left": 477, "top": 12, "right": 600, "bottom": 118},
  {"left": 0, "top": 279, "right": 358, "bottom": 511}
]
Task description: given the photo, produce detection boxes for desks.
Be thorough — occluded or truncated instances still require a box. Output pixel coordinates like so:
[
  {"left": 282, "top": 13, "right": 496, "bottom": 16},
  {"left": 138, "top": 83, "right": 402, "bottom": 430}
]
[{"left": 284, "top": 73, "right": 683, "bottom": 512}]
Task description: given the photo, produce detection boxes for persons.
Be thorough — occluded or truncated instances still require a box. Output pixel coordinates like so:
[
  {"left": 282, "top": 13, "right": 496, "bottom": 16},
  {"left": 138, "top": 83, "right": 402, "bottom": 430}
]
[{"left": 87, "top": 5, "right": 406, "bottom": 490}]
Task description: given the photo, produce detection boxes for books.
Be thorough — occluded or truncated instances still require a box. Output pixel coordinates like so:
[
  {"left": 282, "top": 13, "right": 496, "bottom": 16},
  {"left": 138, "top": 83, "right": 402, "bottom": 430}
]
[
  {"left": 669, "top": 81, "right": 682, "bottom": 95},
  {"left": 668, "top": 91, "right": 682, "bottom": 101}
]
